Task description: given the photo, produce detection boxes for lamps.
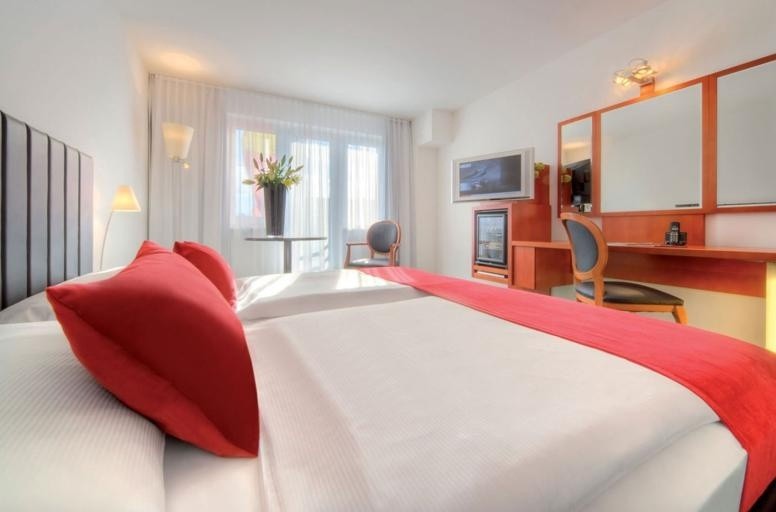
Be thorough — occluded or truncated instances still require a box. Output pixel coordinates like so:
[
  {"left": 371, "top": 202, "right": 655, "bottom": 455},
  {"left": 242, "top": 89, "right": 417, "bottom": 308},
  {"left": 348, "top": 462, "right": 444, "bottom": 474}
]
[
  {"left": 614, "top": 58, "right": 656, "bottom": 86},
  {"left": 161, "top": 123, "right": 193, "bottom": 161},
  {"left": 99, "top": 186, "right": 141, "bottom": 272}
]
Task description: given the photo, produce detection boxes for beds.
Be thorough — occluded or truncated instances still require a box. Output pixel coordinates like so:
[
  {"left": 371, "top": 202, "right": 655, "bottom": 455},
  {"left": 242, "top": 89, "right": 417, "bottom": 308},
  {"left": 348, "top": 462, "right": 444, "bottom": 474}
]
[{"left": 0, "top": 112, "right": 776, "bottom": 512}]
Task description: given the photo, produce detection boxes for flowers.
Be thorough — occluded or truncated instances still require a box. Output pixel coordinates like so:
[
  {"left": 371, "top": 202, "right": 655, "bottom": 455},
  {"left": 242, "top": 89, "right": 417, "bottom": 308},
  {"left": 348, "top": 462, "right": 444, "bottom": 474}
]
[{"left": 242, "top": 153, "right": 305, "bottom": 191}]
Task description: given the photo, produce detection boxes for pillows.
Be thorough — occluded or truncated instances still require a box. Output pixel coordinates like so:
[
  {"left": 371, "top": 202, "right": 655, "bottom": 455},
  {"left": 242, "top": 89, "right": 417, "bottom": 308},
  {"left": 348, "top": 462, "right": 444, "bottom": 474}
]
[
  {"left": 0, "top": 266, "right": 128, "bottom": 328},
  {"left": 45, "top": 241, "right": 259, "bottom": 458},
  {"left": 0, "top": 322, "right": 169, "bottom": 512},
  {"left": 173, "top": 241, "right": 234, "bottom": 312}
]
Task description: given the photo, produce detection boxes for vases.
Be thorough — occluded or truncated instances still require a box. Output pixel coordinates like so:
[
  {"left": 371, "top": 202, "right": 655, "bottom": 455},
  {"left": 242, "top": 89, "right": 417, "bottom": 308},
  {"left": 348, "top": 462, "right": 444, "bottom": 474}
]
[{"left": 262, "top": 180, "right": 287, "bottom": 236}]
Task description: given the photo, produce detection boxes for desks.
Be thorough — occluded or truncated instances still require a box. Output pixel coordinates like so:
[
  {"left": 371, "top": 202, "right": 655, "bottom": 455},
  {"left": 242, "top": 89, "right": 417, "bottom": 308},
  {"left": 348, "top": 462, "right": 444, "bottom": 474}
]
[{"left": 246, "top": 236, "right": 326, "bottom": 273}]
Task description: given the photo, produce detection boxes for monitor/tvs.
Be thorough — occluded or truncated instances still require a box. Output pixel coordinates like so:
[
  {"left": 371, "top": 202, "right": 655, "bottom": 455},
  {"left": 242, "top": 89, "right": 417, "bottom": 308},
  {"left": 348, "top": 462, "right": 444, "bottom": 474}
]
[{"left": 564, "top": 159, "right": 591, "bottom": 204}]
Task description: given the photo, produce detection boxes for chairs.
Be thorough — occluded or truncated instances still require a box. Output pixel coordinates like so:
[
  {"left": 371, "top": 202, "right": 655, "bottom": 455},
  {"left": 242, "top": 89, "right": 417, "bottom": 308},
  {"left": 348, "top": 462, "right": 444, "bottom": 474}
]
[
  {"left": 344, "top": 221, "right": 400, "bottom": 268},
  {"left": 560, "top": 212, "right": 689, "bottom": 325}
]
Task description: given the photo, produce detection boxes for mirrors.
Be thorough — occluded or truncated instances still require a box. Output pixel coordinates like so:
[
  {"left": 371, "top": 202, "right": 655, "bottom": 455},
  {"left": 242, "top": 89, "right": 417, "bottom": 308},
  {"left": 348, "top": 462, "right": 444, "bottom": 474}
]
[{"left": 557, "top": 113, "right": 596, "bottom": 217}]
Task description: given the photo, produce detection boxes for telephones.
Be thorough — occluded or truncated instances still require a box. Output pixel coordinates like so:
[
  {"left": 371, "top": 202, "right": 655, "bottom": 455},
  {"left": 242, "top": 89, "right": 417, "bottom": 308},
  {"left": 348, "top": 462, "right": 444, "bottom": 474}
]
[{"left": 664, "top": 221, "right": 687, "bottom": 245}]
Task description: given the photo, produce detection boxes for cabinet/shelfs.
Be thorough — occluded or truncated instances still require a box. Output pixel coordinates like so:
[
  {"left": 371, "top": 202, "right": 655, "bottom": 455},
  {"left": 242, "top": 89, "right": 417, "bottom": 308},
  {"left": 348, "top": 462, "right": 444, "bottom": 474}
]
[
  {"left": 512, "top": 246, "right": 572, "bottom": 295},
  {"left": 470, "top": 202, "right": 552, "bottom": 285}
]
